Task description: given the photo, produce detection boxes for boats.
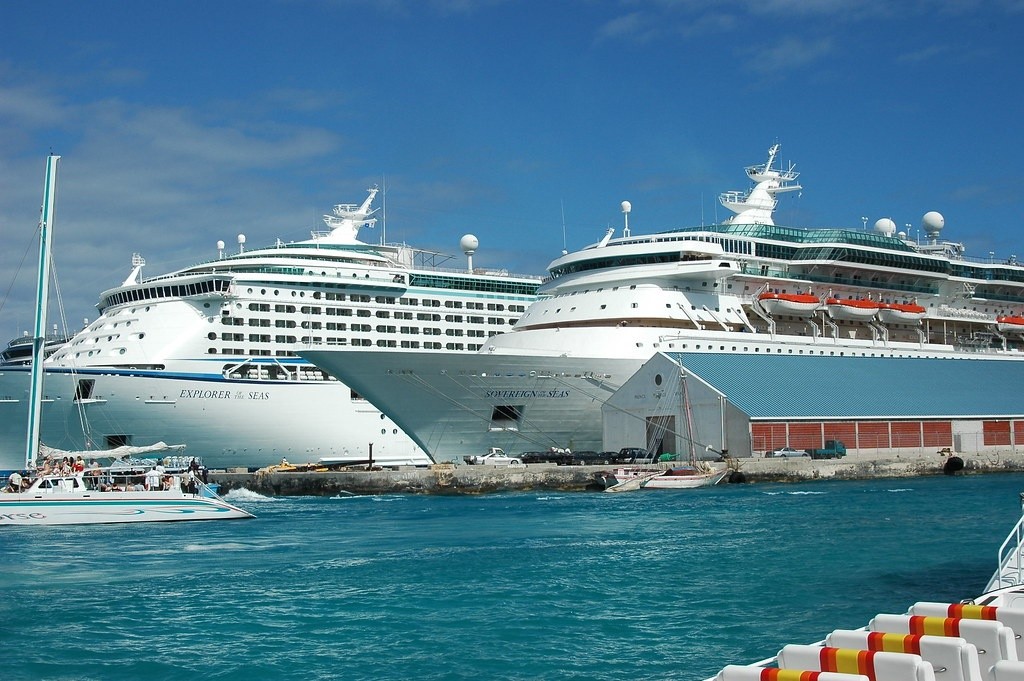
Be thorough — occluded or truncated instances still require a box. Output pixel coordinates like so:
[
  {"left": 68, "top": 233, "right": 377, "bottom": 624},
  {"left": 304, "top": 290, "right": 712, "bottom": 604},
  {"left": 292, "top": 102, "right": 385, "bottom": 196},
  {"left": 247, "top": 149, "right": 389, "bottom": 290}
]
[{"left": 0, "top": 443, "right": 259, "bottom": 526}]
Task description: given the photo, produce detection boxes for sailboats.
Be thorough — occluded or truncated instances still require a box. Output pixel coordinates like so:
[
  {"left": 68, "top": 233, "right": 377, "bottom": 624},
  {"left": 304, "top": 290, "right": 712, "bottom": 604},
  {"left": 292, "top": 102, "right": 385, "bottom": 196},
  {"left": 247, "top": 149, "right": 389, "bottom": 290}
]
[{"left": 599, "top": 354, "right": 731, "bottom": 492}]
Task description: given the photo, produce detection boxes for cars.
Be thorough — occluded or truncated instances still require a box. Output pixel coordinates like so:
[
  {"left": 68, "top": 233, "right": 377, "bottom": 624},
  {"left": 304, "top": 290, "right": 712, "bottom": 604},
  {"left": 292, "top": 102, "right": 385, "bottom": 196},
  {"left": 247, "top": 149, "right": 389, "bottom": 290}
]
[
  {"left": 514, "top": 451, "right": 546, "bottom": 464},
  {"left": 615, "top": 447, "right": 658, "bottom": 464},
  {"left": 473, "top": 452, "right": 523, "bottom": 465},
  {"left": 565, "top": 450, "right": 598, "bottom": 465},
  {"left": 765, "top": 447, "right": 808, "bottom": 457},
  {"left": 591, "top": 451, "right": 620, "bottom": 465},
  {"left": 546, "top": 450, "right": 572, "bottom": 466}
]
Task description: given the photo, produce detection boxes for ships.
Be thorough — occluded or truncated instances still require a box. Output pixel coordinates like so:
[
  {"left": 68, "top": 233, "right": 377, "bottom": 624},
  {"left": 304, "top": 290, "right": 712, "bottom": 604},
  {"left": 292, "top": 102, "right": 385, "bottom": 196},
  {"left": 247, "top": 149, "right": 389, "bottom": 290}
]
[
  {"left": 0, "top": 184, "right": 550, "bottom": 471},
  {"left": 297, "top": 145, "right": 1024, "bottom": 465}
]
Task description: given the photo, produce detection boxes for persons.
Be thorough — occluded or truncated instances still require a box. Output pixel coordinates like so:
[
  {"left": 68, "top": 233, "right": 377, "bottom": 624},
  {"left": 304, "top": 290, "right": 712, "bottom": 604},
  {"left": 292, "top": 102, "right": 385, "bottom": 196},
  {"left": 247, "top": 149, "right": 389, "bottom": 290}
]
[
  {"left": 35, "top": 456, "right": 86, "bottom": 481},
  {"left": 6, "top": 469, "right": 33, "bottom": 494},
  {"left": 282, "top": 456, "right": 334, "bottom": 473},
  {"left": 88, "top": 458, "right": 224, "bottom": 494}
]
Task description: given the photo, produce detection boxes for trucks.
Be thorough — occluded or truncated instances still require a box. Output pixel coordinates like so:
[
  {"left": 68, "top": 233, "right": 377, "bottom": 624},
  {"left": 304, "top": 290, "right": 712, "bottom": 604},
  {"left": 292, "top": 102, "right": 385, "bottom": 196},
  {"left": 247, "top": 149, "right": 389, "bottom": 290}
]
[
  {"left": 804, "top": 438, "right": 847, "bottom": 460},
  {"left": 462, "top": 447, "right": 509, "bottom": 466}
]
